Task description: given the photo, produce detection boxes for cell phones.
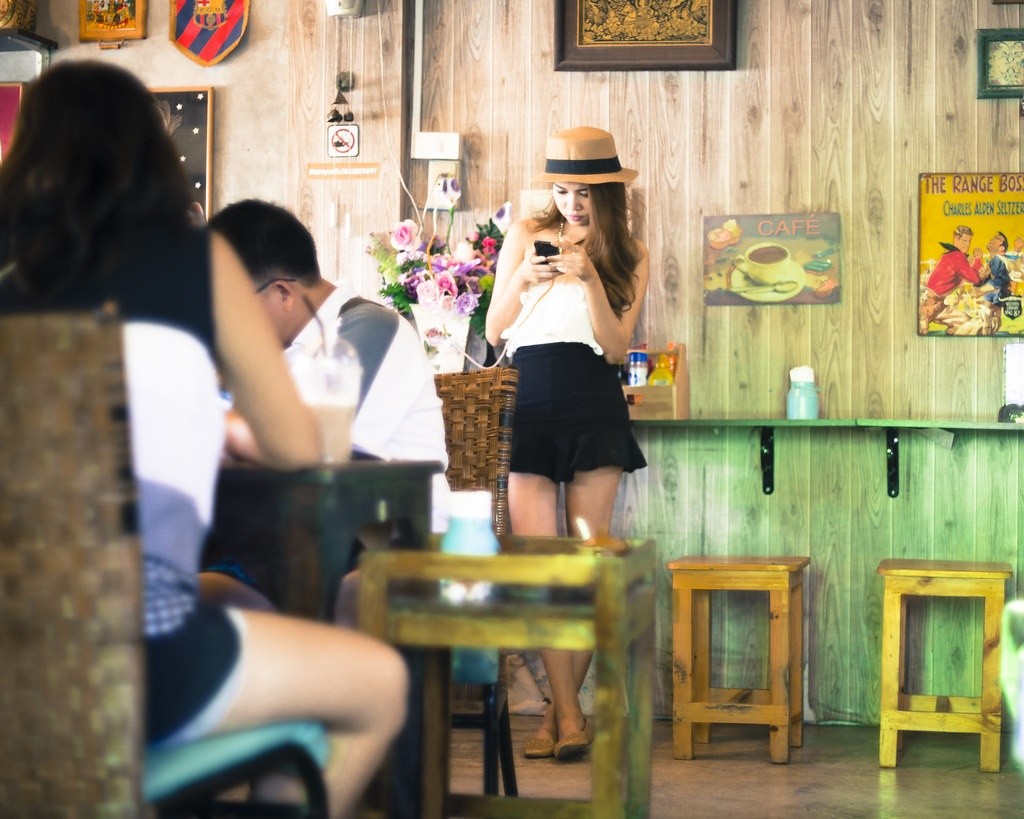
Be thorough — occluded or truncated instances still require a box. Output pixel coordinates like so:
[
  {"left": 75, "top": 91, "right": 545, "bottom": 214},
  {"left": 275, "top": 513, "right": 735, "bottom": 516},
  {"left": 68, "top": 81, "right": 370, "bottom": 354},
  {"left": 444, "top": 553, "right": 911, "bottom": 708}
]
[{"left": 534, "top": 241, "right": 560, "bottom": 264}]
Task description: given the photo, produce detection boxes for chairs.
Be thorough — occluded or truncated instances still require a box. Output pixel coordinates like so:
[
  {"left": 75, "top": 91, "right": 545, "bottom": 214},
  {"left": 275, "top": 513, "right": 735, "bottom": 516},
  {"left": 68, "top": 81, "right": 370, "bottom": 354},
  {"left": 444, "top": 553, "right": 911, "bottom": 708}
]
[{"left": 0, "top": 305, "right": 331, "bottom": 818}]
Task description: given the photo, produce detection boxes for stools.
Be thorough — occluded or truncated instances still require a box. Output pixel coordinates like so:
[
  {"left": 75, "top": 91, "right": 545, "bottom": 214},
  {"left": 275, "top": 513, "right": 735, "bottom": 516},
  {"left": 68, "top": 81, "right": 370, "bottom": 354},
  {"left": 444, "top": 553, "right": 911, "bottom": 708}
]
[
  {"left": 349, "top": 540, "right": 654, "bottom": 818},
  {"left": 667, "top": 556, "right": 810, "bottom": 764},
  {"left": 877, "top": 558, "right": 1013, "bottom": 774}
]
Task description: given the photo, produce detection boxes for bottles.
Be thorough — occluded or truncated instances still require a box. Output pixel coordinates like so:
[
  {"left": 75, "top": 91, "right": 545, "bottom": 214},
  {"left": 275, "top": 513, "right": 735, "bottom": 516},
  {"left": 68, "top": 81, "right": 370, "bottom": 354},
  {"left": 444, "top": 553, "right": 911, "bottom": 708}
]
[
  {"left": 786, "top": 367, "right": 821, "bottom": 421},
  {"left": 613, "top": 342, "right": 679, "bottom": 386}
]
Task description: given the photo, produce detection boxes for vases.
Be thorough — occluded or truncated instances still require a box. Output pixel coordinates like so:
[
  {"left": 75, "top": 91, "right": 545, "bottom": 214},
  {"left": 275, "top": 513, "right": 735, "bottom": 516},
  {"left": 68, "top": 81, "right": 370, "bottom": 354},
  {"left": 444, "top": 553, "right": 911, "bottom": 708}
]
[{"left": 408, "top": 298, "right": 475, "bottom": 375}]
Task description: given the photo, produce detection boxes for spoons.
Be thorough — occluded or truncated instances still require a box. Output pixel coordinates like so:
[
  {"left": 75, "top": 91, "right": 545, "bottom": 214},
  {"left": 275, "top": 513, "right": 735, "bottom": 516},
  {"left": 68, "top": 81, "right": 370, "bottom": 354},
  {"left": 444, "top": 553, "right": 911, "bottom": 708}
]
[{"left": 731, "top": 281, "right": 797, "bottom": 294}]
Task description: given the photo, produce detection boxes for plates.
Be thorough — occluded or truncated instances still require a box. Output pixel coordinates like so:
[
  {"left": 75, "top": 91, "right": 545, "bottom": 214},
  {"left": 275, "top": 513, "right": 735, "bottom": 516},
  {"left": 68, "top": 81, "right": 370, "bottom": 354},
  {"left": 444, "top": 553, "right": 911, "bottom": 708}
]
[{"left": 730, "top": 262, "right": 806, "bottom": 301}]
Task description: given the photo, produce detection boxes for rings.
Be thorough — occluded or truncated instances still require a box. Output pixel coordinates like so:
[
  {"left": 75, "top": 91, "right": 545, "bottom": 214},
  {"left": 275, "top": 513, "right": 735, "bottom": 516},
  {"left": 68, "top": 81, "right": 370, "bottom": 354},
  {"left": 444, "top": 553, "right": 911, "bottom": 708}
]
[{"left": 574, "top": 244, "right": 579, "bottom": 254}]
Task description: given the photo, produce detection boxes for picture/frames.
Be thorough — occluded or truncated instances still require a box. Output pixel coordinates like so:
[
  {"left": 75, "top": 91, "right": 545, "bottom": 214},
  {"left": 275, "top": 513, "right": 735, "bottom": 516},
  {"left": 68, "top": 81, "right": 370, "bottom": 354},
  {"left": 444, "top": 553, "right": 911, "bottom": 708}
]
[
  {"left": 550, "top": 0, "right": 738, "bottom": 68},
  {"left": 465, "top": 309, "right": 489, "bottom": 374},
  {"left": 977, "top": 28, "right": 1024, "bottom": 97},
  {"left": 148, "top": 87, "right": 215, "bottom": 221},
  {"left": 75, "top": 0, "right": 148, "bottom": 43}
]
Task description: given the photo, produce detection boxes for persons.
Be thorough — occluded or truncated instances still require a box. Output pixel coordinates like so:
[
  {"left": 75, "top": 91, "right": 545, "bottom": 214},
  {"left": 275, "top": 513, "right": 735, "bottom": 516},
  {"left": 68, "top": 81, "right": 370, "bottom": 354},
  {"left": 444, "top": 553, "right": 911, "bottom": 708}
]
[
  {"left": 0, "top": 61, "right": 411, "bottom": 819},
  {"left": 484, "top": 128, "right": 646, "bottom": 759},
  {"left": 200, "top": 199, "right": 453, "bottom": 534}
]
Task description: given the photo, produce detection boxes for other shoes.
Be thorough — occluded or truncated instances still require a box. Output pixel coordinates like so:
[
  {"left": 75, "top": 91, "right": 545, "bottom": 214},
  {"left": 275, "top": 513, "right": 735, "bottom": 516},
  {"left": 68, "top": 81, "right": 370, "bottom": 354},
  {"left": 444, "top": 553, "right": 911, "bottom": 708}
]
[
  {"left": 524, "top": 737, "right": 555, "bottom": 757},
  {"left": 554, "top": 716, "right": 596, "bottom": 760}
]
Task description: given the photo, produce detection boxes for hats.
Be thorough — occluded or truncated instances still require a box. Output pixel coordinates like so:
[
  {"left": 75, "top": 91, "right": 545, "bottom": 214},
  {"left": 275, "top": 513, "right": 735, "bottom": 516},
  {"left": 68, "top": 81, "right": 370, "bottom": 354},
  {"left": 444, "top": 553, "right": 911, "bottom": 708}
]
[{"left": 545, "top": 128, "right": 639, "bottom": 184}]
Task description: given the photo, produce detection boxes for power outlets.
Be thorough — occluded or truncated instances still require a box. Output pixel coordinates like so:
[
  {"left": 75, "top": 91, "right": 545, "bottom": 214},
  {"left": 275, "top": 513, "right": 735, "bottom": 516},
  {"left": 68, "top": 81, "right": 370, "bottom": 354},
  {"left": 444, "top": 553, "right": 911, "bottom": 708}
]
[{"left": 426, "top": 160, "right": 464, "bottom": 212}]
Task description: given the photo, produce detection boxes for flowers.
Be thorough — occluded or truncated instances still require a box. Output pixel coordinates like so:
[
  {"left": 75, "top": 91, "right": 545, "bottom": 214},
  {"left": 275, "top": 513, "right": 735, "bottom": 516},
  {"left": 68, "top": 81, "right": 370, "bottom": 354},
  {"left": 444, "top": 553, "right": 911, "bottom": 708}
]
[{"left": 362, "top": 172, "right": 508, "bottom": 340}]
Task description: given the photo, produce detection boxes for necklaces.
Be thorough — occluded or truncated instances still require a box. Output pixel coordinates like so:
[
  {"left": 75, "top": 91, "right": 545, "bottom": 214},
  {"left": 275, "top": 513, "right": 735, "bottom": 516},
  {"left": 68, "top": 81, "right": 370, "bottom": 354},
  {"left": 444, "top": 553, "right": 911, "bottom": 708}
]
[{"left": 556, "top": 219, "right": 602, "bottom": 260}]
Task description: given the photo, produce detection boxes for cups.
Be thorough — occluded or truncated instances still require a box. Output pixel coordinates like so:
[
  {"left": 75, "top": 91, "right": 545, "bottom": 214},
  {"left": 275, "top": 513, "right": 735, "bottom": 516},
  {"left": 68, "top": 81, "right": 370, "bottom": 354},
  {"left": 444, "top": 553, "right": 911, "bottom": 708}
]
[{"left": 734, "top": 243, "right": 790, "bottom": 285}]
